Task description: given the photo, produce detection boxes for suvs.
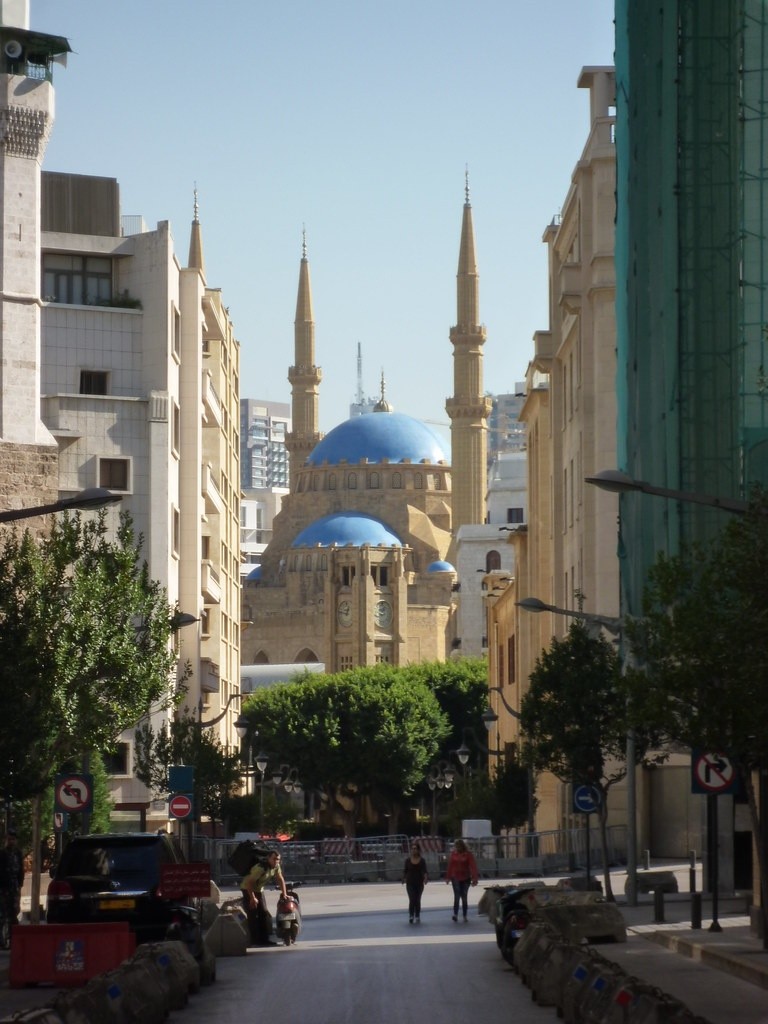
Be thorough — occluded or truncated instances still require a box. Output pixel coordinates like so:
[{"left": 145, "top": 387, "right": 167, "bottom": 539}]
[{"left": 44, "top": 828, "right": 189, "bottom": 944}]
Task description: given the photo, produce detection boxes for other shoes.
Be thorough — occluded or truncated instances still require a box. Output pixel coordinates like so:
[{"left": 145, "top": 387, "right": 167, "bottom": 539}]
[
  {"left": 409, "top": 917, "right": 413, "bottom": 923},
  {"left": 261, "top": 940, "right": 276, "bottom": 947},
  {"left": 464, "top": 918, "right": 468, "bottom": 921},
  {"left": 415, "top": 919, "right": 420, "bottom": 924},
  {"left": 452, "top": 916, "right": 458, "bottom": 922}
]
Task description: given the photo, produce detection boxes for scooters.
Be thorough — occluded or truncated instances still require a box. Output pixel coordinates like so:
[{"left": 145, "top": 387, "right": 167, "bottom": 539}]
[{"left": 275, "top": 881, "right": 307, "bottom": 946}]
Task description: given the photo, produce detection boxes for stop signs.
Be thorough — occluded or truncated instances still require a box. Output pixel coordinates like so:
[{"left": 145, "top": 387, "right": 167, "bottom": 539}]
[{"left": 169, "top": 794, "right": 193, "bottom": 820}]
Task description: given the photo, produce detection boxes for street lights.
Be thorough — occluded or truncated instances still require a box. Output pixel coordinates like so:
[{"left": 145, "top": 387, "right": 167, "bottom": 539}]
[
  {"left": 481, "top": 705, "right": 500, "bottom": 837},
  {"left": 515, "top": 598, "right": 637, "bottom": 907},
  {"left": 426, "top": 758, "right": 455, "bottom": 836},
  {"left": 29, "top": 613, "right": 202, "bottom": 924},
  {"left": 255, "top": 750, "right": 269, "bottom": 834},
  {"left": 455, "top": 685, "right": 538, "bottom": 857},
  {"left": 196, "top": 694, "right": 250, "bottom": 835}
]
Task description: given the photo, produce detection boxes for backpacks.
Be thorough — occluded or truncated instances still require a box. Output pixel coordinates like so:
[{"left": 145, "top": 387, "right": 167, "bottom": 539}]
[{"left": 227, "top": 840, "right": 268, "bottom": 887}]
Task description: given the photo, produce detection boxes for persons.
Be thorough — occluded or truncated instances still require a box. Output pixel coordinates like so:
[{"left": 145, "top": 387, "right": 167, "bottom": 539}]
[
  {"left": 445, "top": 839, "right": 477, "bottom": 923},
  {"left": 240, "top": 849, "right": 288, "bottom": 947},
  {"left": 402, "top": 844, "right": 428, "bottom": 924}
]
